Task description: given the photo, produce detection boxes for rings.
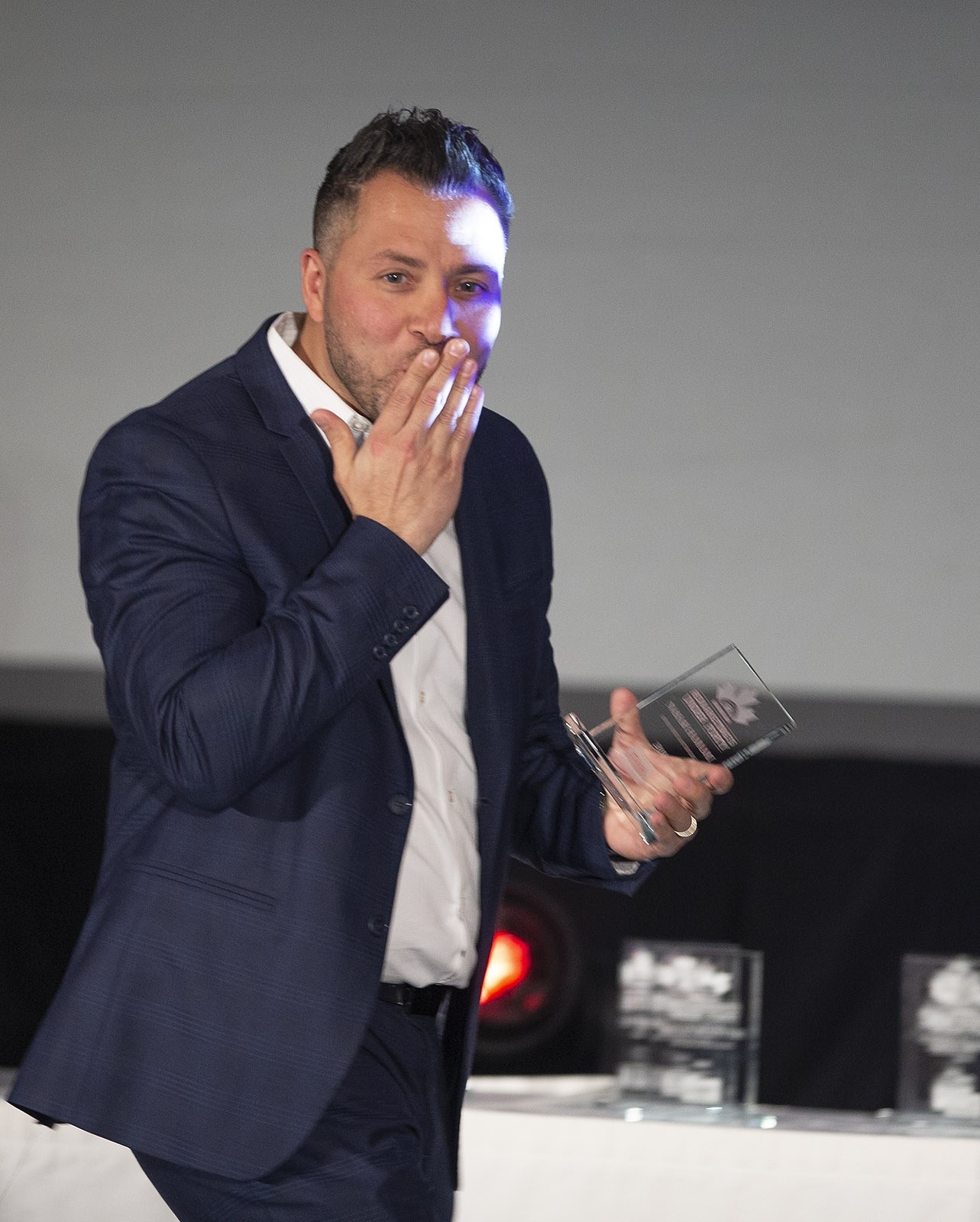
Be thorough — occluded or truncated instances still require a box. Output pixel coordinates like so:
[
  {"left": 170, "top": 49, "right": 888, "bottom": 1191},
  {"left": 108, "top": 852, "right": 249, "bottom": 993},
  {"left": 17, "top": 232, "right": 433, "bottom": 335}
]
[{"left": 671, "top": 816, "right": 697, "bottom": 838}]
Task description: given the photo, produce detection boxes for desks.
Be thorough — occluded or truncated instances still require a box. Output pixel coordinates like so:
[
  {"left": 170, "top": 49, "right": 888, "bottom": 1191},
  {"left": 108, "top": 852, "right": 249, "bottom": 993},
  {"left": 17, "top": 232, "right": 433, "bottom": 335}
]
[{"left": 0, "top": 1047, "right": 980, "bottom": 1222}]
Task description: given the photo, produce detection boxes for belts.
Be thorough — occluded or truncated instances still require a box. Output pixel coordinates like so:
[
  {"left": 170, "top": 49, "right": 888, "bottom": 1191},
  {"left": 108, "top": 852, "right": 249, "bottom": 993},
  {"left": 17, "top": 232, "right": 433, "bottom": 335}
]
[{"left": 378, "top": 982, "right": 474, "bottom": 1020}]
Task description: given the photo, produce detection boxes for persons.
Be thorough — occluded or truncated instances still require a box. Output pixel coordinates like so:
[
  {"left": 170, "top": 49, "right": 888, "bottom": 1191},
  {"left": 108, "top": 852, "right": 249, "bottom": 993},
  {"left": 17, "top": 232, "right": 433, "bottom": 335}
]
[{"left": 7, "top": 106, "right": 734, "bottom": 1222}]
